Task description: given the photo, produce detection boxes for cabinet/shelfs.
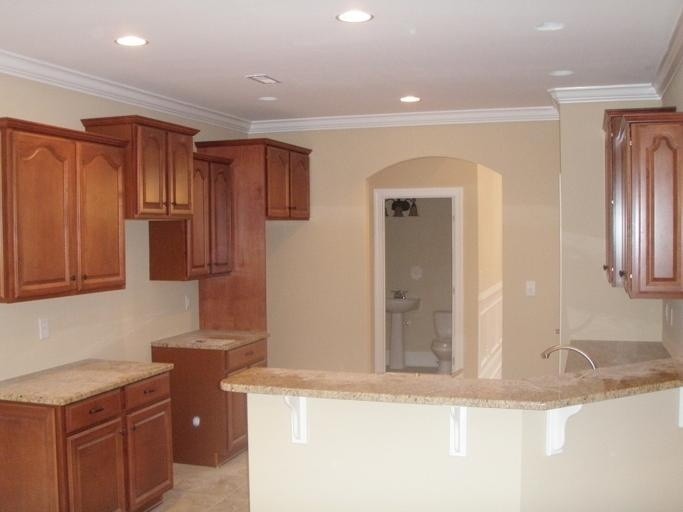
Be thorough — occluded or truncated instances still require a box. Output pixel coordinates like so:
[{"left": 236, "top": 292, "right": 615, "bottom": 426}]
[
  {"left": 224, "top": 336, "right": 267, "bottom": 457},
  {"left": 2, "top": 116, "right": 128, "bottom": 302},
  {"left": 55, "top": 372, "right": 174, "bottom": 512},
  {"left": 603, "top": 107, "right": 676, "bottom": 287},
  {"left": 187, "top": 159, "right": 234, "bottom": 279},
  {"left": 136, "top": 115, "right": 194, "bottom": 219},
  {"left": 265, "top": 146, "right": 309, "bottom": 220},
  {"left": 620, "top": 113, "right": 683, "bottom": 299}
]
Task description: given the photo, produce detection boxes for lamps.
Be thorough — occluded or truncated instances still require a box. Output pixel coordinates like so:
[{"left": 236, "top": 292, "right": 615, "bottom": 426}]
[{"left": 385, "top": 198, "right": 418, "bottom": 218}]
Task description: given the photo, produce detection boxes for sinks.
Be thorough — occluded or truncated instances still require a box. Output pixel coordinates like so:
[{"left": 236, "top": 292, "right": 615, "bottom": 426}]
[{"left": 386, "top": 297, "right": 418, "bottom": 313}]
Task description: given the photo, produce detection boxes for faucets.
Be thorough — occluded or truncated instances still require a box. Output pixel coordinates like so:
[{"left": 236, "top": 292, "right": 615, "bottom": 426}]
[
  {"left": 541, "top": 344, "right": 597, "bottom": 372},
  {"left": 394, "top": 289, "right": 407, "bottom": 300}
]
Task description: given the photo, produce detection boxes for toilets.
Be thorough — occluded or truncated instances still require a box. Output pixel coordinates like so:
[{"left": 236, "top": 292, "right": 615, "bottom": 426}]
[{"left": 432, "top": 310, "right": 451, "bottom": 376}]
